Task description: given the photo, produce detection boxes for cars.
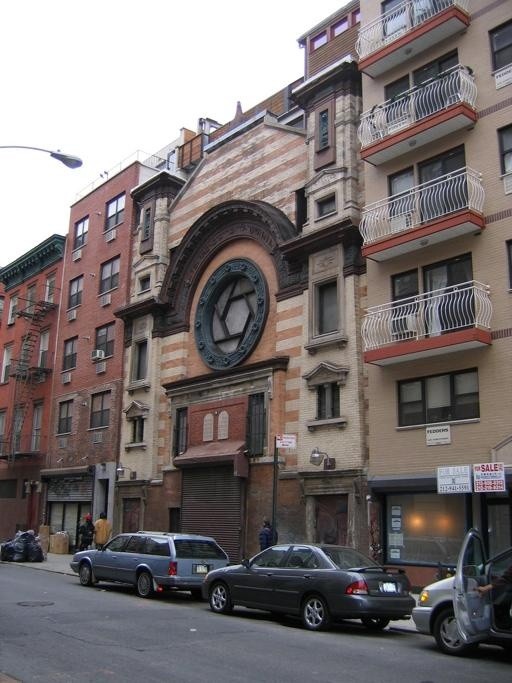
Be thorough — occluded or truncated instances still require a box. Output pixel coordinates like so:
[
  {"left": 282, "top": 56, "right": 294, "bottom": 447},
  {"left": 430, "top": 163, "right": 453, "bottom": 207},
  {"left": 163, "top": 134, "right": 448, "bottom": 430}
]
[
  {"left": 412, "top": 529, "right": 511, "bottom": 655},
  {"left": 70, "top": 530, "right": 230, "bottom": 597},
  {"left": 201, "top": 542, "right": 417, "bottom": 632}
]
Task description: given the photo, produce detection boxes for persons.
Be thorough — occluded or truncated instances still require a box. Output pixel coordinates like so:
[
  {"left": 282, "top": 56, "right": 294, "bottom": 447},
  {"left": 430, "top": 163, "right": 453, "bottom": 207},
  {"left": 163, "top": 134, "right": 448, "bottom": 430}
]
[
  {"left": 476, "top": 562, "right": 512, "bottom": 592},
  {"left": 78, "top": 514, "right": 94, "bottom": 551},
  {"left": 94, "top": 510, "right": 111, "bottom": 550},
  {"left": 258, "top": 515, "right": 278, "bottom": 551}
]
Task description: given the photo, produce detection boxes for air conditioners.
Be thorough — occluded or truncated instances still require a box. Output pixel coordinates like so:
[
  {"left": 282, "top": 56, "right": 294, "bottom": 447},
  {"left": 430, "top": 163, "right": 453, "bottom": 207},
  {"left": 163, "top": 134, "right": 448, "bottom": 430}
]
[
  {"left": 91, "top": 350, "right": 105, "bottom": 360},
  {"left": 96, "top": 362, "right": 106, "bottom": 374},
  {"left": 72, "top": 250, "right": 82, "bottom": 261},
  {"left": 67, "top": 310, "right": 76, "bottom": 321},
  {"left": 61, "top": 373, "right": 71, "bottom": 383},
  {"left": 390, "top": 314, "right": 416, "bottom": 336},
  {"left": 104, "top": 230, "right": 116, "bottom": 243},
  {"left": 101, "top": 294, "right": 111, "bottom": 306}
]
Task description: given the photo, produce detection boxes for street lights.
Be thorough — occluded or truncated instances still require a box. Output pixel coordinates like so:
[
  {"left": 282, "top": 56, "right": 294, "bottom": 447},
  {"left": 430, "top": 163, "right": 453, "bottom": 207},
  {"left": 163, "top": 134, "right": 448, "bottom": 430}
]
[{"left": 2, "top": 145, "right": 82, "bottom": 171}]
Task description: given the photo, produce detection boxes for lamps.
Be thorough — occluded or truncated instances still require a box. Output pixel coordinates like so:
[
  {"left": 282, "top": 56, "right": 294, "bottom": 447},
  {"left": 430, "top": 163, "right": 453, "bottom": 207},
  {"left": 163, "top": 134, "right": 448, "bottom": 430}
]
[{"left": 309, "top": 447, "right": 336, "bottom": 470}]
